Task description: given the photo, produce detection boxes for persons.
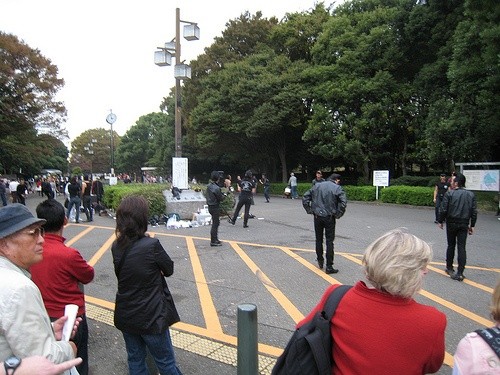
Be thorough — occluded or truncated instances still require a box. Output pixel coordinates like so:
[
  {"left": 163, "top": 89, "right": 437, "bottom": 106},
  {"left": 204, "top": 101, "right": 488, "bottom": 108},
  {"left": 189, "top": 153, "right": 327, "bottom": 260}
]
[
  {"left": 226, "top": 170, "right": 255, "bottom": 228},
  {"left": 29, "top": 198, "right": 95, "bottom": 375},
  {"left": 0, "top": 164, "right": 255, "bottom": 224},
  {"left": 0, "top": 350, "right": 83, "bottom": 374},
  {"left": 438, "top": 171, "right": 477, "bottom": 281},
  {"left": 294, "top": 230, "right": 445, "bottom": 375},
  {"left": 287, "top": 173, "right": 300, "bottom": 199},
  {"left": 0, "top": 202, "right": 84, "bottom": 375},
  {"left": 452, "top": 279, "right": 500, "bottom": 375},
  {"left": 206, "top": 172, "right": 225, "bottom": 246},
  {"left": 110, "top": 195, "right": 183, "bottom": 375},
  {"left": 259, "top": 174, "right": 271, "bottom": 203},
  {"left": 301, "top": 173, "right": 347, "bottom": 274},
  {"left": 433, "top": 174, "right": 451, "bottom": 224},
  {"left": 312, "top": 171, "right": 326, "bottom": 185}
]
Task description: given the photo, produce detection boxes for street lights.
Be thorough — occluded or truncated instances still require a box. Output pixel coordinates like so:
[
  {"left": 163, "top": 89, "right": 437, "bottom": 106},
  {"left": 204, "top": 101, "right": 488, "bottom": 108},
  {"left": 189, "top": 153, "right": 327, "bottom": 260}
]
[{"left": 153, "top": 8, "right": 208, "bottom": 219}]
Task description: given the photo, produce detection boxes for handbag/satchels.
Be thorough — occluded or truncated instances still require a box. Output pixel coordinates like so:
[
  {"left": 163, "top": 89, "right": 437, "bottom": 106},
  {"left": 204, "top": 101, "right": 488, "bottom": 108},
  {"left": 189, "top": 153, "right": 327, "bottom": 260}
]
[
  {"left": 271, "top": 285, "right": 353, "bottom": 375},
  {"left": 64, "top": 198, "right": 70, "bottom": 208}
]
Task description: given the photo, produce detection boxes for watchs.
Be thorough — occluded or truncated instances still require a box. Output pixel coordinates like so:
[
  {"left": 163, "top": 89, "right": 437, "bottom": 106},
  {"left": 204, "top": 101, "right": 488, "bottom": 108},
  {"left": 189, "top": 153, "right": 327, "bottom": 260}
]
[{"left": 2, "top": 354, "right": 23, "bottom": 375}]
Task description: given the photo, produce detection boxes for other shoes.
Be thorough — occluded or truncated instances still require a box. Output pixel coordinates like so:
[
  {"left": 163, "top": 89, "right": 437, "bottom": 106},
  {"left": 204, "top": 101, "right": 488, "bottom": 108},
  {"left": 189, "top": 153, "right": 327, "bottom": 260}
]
[
  {"left": 319, "top": 263, "right": 324, "bottom": 268},
  {"left": 228, "top": 220, "right": 234, "bottom": 225},
  {"left": 326, "top": 268, "right": 338, "bottom": 274},
  {"left": 210, "top": 242, "right": 222, "bottom": 247},
  {"left": 76, "top": 222, "right": 79, "bottom": 223},
  {"left": 86, "top": 219, "right": 90, "bottom": 222},
  {"left": 457, "top": 274, "right": 464, "bottom": 281},
  {"left": 216, "top": 240, "right": 221, "bottom": 243},
  {"left": 446, "top": 269, "right": 453, "bottom": 276},
  {"left": 243, "top": 225, "right": 248, "bottom": 228}
]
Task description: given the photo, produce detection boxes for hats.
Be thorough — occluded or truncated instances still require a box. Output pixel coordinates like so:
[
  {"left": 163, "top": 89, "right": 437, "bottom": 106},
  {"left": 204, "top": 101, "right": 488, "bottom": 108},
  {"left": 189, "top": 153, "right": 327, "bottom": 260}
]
[{"left": 0, "top": 203, "right": 47, "bottom": 239}]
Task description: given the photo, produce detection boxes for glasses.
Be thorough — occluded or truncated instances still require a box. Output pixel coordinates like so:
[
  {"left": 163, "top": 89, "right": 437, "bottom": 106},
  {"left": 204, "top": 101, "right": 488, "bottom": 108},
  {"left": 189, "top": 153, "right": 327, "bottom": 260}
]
[{"left": 18, "top": 227, "right": 44, "bottom": 240}]
